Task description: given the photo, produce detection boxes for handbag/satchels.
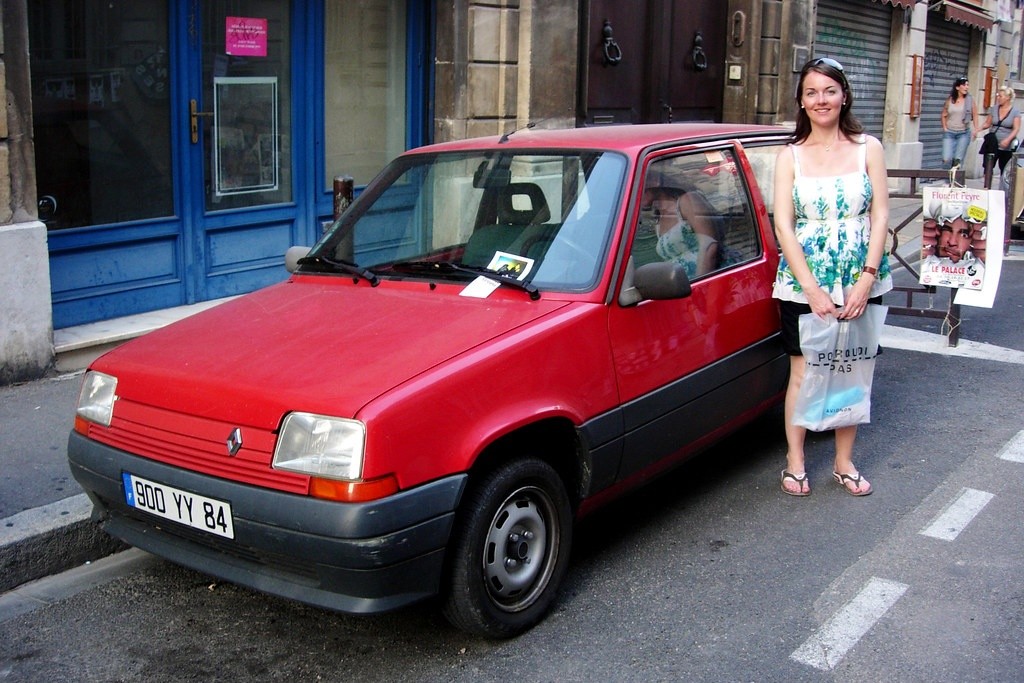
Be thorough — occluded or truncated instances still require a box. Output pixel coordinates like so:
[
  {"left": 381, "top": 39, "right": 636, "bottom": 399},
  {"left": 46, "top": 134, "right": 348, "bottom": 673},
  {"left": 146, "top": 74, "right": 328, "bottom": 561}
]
[
  {"left": 791, "top": 304, "right": 888, "bottom": 432},
  {"left": 979, "top": 132, "right": 999, "bottom": 154}
]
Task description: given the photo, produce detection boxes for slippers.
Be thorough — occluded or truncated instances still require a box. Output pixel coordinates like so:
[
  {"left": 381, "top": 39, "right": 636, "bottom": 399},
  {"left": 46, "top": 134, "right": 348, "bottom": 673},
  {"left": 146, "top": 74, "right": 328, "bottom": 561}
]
[
  {"left": 781, "top": 469, "right": 811, "bottom": 495},
  {"left": 833, "top": 471, "right": 873, "bottom": 495}
]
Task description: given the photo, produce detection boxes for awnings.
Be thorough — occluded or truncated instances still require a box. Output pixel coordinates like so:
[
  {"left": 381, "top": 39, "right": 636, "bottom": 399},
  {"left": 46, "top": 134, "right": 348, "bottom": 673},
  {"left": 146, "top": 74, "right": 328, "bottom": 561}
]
[{"left": 872, "top": 0, "right": 993, "bottom": 33}]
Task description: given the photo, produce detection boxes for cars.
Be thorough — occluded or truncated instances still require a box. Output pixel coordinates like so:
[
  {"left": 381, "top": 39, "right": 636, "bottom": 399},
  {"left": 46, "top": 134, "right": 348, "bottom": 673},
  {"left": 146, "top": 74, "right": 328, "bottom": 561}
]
[{"left": 64, "top": 122, "right": 810, "bottom": 643}]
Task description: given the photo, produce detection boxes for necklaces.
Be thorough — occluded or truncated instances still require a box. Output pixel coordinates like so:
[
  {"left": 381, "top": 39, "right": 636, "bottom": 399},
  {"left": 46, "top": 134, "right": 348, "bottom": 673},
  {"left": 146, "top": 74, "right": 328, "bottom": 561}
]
[{"left": 825, "top": 145, "right": 830, "bottom": 151}]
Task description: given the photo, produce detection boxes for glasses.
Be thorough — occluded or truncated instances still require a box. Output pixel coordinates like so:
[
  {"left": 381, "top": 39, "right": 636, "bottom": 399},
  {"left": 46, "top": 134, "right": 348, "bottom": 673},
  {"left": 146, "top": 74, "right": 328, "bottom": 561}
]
[{"left": 800, "top": 58, "right": 844, "bottom": 74}]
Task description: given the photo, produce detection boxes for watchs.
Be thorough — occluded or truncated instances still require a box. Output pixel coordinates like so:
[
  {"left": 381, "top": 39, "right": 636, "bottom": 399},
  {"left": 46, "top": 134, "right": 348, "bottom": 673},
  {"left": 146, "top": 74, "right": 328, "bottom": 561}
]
[{"left": 863, "top": 265, "right": 881, "bottom": 279}]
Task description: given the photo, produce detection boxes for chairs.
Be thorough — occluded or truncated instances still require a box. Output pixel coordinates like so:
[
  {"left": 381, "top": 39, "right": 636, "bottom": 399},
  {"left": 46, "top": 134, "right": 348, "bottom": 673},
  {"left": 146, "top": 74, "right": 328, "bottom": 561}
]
[{"left": 462, "top": 183, "right": 665, "bottom": 284}]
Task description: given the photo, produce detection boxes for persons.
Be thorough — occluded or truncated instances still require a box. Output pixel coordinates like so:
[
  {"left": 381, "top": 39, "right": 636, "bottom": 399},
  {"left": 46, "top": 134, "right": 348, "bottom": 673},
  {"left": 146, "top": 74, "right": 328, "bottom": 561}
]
[
  {"left": 972, "top": 84, "right": 1022, "bottom": 191},
  {"left": 771, "top": 59, "right": 889, "bottom": 496},
  {"left": 941, "top": 77, "right": 978, "bottom": 185},
  {"left": 923, "top": 191, "right": 988, "bottom": 265},
  {"left": 643, "top": 172, "right": 743, "bottom": 280}
]
